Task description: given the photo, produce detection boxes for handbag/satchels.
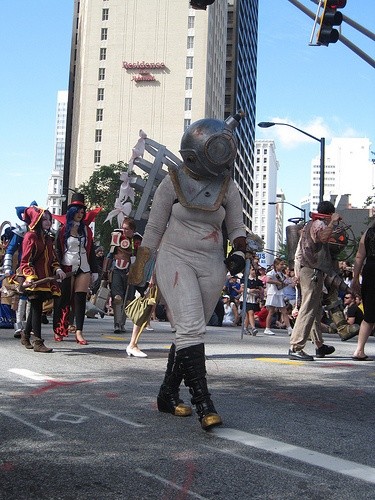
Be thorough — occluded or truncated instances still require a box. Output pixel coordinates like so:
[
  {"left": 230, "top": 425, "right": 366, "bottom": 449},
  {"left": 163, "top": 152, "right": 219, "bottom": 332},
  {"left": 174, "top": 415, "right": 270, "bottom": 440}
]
[{"left": 124, "top": 286, "right": 155, "bottom": 327}]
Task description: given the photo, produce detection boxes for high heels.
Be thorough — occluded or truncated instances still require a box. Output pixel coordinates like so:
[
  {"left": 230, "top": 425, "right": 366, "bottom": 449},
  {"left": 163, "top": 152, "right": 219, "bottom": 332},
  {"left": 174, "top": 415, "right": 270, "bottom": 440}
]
[
  {"left": 54, "top": 334, "right": 63, "bottom": 341},
  {"left": 126, "top": 346, "right": 147, "bottom": 357},
  {"left": 75, "top": 336, "right": 87, "bottom": 345}
]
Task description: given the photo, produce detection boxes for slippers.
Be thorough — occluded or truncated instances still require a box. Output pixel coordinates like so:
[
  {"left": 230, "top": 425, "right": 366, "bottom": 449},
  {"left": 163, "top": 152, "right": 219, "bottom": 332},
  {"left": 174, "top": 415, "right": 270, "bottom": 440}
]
[{"left": 352, "top": 355, "right": 373, "bottom": 361}]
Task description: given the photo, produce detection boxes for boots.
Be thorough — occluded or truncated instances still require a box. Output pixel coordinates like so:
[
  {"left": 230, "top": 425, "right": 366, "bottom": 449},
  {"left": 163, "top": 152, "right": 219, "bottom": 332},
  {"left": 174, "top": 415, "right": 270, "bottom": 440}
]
[
  {"left": 21, "top": 332, "right": 34, "bottom": 349},
  {"left": 33, "top": 338, "right": 52, "bottom": 352},
  {"left": 175, "top": 343, "right": 223, "bottom": 428},
  {"left": 157, "top": 342, "right": 193, "bottom": 416}
]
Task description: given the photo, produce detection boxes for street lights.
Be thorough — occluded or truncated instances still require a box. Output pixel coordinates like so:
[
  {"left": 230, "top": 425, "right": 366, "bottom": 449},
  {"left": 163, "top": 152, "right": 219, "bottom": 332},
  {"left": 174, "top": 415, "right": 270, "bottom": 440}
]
[
  {"left": 257, "top": 121, "right": 325, "bottom": 202},
  {"left": 268, "top": 201, "right": 306, "bottom": 226}
]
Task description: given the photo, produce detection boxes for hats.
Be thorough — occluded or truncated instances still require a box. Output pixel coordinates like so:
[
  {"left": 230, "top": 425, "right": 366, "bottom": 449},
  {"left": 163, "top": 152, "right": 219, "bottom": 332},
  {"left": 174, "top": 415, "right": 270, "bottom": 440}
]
[
  {"left": 223, "top": 295, "right": 230, "bottom": 299},
  {"left": 23, "top": 205, "right": 45, "bottom": 230},
  {"left": 68, "top": 193, "right": 87, "bottom": 209},
  {"left": 227, "top": 272, "right": 243, "bottom": 279}
]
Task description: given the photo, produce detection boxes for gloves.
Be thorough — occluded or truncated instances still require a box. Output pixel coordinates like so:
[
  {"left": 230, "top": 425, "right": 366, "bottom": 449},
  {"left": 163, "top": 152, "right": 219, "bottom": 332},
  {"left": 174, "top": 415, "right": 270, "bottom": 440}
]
[{"left": 224, "top": 252, "right": 246, "bottom": 276}]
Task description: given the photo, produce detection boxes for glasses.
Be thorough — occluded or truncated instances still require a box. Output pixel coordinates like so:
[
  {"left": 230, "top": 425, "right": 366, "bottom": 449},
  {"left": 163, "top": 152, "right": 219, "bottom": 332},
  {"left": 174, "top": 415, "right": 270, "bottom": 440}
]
[{"left": 344, "top": 297, "right": 351, "bottom": 299}]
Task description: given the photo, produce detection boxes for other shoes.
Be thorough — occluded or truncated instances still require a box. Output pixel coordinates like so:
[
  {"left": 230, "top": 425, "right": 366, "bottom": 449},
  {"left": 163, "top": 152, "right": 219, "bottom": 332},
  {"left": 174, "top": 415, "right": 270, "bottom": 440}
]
[
  {"left": 14, "top": 331, "right": 21, "bottom": 339},
  {"left": 338, "top": 325, "right": 359, "bottom": 342},
  {"left": 252, "top": 328, "right": 258, "bottom": 336},
  {"left": 146, "top": 327, "right": 154, "bottom": 331},
  {"left": 288, "top": 329, "right": 294, "bottom": 336},
  {"left": 264, "top": 330, "right": 275, "bottom": 335},
  {"left": 114, "top": 329, "right": 125, "bottom": 334},
  {"left": 316, "top": 345, "right": 335, "bottom": 358},
  {"left": 288, "top": 349, "right": 313, "bottom": 361},
  {"left": 244, "top": 329, "right": 250, "bottom": 335}
]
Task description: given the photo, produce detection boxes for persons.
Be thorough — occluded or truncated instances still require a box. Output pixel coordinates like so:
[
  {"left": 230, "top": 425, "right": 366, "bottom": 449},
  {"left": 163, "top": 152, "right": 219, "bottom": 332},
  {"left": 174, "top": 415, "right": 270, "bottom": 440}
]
[
  {"left": 288, "top": 201, "right": 342, "bottom": 361},
  {"left": 0, "top": 197, "right": 176, "bottom": 353},
  {"left": 131, "top": 124, "right": 246, "bottom": 429},
  {"left": 126, "top": 264, "right": 157, "bottom": 358},
  {"left": 103, "top": 218, "right": 144, "bottom": 334},
  {"left": 350, "top": 221, "right": 375, "bottom": 361},
  {"left": 264, "top": 258, "right": 293, "bottom": 336},
  {"left": 207, "top": 261, "right": 374, "bottom": 342},
  {"left": 243, "top": 264, "right": 264, "bottom": 336}
]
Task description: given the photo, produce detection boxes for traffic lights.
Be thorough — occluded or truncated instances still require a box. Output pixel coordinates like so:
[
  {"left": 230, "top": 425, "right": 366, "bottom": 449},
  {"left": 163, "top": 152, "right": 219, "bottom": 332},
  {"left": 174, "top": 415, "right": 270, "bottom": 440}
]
[{"left": 316, "top": 0, "right": 347, "bottom": 47}]
